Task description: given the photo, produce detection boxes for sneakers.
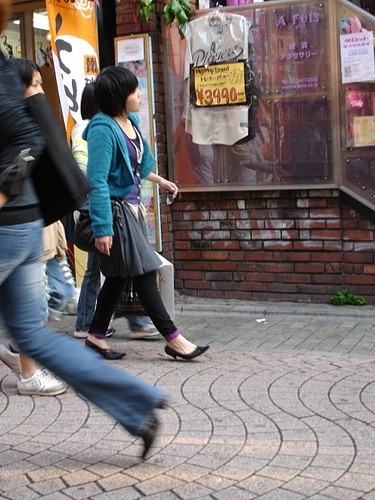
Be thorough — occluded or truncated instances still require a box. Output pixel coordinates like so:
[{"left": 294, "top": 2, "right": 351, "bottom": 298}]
[
  {"left": 128, "top": 323, "right": 161, "bottom": 339},
  {"left": 74, "top": 324, "right": 115, "bottom": 338},
  {"left": 17, "top": 369, "right": 69, "bottom": 397},
  {"left": 0, "top": 340, "right": 22, "bottom": 375}
]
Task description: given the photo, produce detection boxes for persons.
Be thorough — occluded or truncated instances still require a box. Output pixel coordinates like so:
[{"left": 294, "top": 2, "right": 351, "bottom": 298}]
[
  {"left": 0, "top": 47, "right": 168, "bottom": 461},
  {"left": 0, "top": 58, "right": 210, "bottom": 396},
  {"left": 172, "top": 93, "right": 329, "bottom": 184}
]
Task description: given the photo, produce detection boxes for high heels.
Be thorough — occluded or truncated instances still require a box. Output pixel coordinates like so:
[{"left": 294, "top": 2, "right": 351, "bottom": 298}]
[
  {"left": 85, "top": 339, "right": 126, "bottom": 360},
  {"left": 164, "top": 345, "right": 210, "bottom": 360},
  {"left": 139, "top": 395, "right": 167, "bottom": 459}
]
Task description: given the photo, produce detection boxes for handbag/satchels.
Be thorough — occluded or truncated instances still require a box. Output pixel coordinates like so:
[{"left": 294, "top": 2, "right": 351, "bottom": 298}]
[
  {"left": 21, "top": 94, "right": 90, "bottom": 226},
  {"left": 72, "top": 208, "right": 118, "bottom": 252}
]
